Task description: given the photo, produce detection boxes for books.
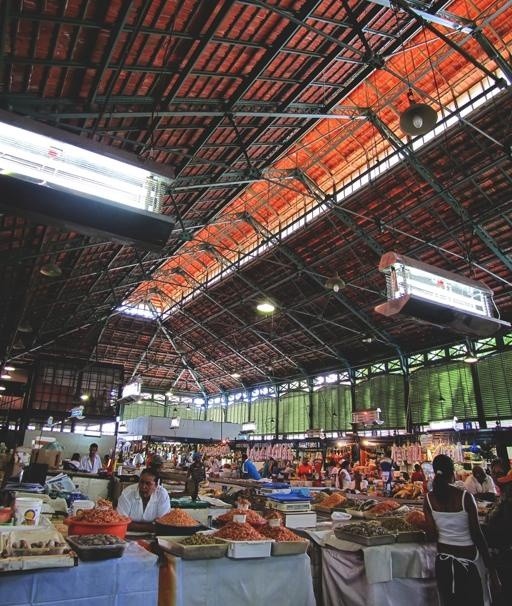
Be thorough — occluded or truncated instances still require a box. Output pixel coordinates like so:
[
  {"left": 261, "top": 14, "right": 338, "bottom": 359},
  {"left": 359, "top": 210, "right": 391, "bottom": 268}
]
[{"left": 3, "top": 473, "right": 77, "bottom": 495}]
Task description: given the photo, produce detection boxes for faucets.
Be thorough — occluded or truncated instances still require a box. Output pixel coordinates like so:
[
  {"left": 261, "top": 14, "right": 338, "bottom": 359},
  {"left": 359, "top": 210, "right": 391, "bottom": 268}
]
[{"left": 14, "top": 498, "right": 43, "bottom": 531}]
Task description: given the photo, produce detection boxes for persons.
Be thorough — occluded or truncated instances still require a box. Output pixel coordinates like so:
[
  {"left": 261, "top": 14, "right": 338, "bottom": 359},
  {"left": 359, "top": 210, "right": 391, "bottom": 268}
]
[
  {"left": 300, "top": 457, "right": 424, "bottom": 498},
  {"left": 68, "top": 443, "right": 114, "bottom": 476},
  {"left": 132, "top": 447, "right": 221, "bottom": 494},
  {"left": 239, "top": 455, "right": 298, "bottom": 480},
  {"left": 116, "top": 471, "right": 170, "bottom": 523},
  {"left": 423, "top": 454, "right": 512, "bottom": 606}
]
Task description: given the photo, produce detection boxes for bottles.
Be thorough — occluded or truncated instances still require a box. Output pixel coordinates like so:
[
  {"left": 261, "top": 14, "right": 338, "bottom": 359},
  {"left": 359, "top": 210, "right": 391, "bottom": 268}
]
[
  {"left": 118, "top": 466, "right": 122, "bottom": 476},
  {"left": 71, "top": 485, "right": 82, "bottom": 512}
]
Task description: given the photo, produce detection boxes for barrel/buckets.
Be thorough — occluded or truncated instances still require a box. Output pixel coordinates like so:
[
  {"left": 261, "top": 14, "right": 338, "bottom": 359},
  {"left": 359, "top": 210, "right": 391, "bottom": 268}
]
[{"left": 18, "top": 469, "right": 25, "bottom": 486}]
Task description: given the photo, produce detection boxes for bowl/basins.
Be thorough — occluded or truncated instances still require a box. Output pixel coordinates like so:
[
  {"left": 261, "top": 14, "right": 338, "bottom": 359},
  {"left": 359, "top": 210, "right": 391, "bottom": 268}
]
[
  {"left": 152, "top": 518, "right": 203, "bottom": 537},
  {"left": 63, "top": 517, "right": 132, "bottom": 539}
]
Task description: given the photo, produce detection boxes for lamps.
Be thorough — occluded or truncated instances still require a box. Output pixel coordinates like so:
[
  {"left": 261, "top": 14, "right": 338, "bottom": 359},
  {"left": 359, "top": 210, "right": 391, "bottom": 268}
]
[
  {"left": 1, "top": 106, "right": 179, "bottom": 256},
  {"left": 393, "top": 9, "right": 442, "bottom": 138},
  {"left": 39, "top": 256, "right": 63, "bottom": 278},
  {"left": 325, "top": 268, "right": 348, "bottom": 294},
  {"left": 369, "top": 251, "right": 510, "bottom": 345}
]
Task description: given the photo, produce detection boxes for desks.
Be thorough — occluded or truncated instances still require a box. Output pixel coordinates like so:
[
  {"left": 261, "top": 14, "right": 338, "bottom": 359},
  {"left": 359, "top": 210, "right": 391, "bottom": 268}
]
[{"left": 1, "top": 467, "right": 495, "bottom": 605}]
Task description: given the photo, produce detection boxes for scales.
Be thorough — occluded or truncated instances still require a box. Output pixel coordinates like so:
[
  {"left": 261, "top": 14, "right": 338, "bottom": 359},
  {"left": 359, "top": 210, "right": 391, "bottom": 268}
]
[{"left": 265, "top": 492, "right": 314, "bottom": 512}]
